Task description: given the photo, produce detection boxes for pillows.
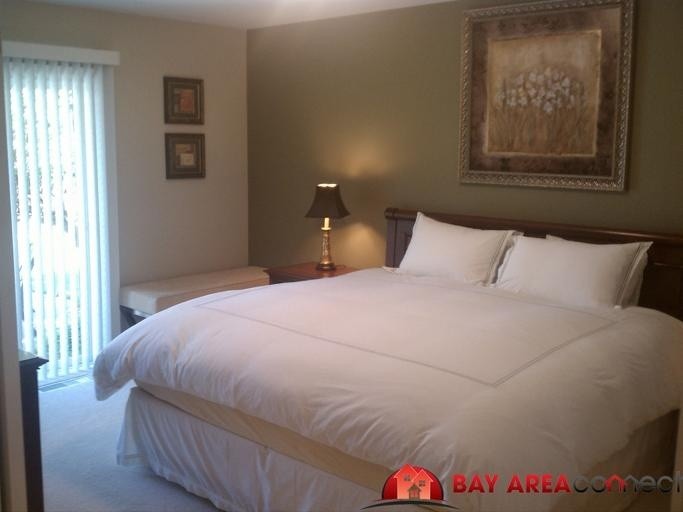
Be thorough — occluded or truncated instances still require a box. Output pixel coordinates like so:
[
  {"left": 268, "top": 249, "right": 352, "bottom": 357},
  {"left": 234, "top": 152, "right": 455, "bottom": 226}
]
[
  {"left": 495, "top": 234, "right": 654, "bottom": 315},
  {"left": 546, "top": 235, "right": 648, "bottom": 306},
  {"left": 399, "top": 211, "right": 518, "bottom": 285}
]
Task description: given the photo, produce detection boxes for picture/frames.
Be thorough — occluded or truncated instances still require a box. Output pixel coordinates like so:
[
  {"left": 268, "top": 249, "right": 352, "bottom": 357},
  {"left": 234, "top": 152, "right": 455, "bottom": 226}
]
[
  {"left": 457, "top": 0, "right": 638, "bottom": 193},
  {"left": 164, "top": 133, "right": 206, "bottom": 180},
  {"left": 163, "top": 76, "right": 206, "bottom": 125}
]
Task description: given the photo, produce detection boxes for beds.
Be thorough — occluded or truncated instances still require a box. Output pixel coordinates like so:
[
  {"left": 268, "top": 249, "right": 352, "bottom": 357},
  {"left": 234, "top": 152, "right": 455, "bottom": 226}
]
[{"left": 93, "top": 206, "right": 683, "bottom": 512}]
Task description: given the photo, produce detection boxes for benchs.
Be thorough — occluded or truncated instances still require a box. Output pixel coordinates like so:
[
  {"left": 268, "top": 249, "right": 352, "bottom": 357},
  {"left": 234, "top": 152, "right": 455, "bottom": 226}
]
[{"left": 120, "top": 265, "right": 271, "bottom": 327}]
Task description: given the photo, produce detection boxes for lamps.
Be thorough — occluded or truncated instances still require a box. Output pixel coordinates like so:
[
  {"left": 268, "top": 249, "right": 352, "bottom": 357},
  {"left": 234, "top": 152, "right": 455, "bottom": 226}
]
[{"left": 304, "top": 183, "right": 352, "bottom": 271}]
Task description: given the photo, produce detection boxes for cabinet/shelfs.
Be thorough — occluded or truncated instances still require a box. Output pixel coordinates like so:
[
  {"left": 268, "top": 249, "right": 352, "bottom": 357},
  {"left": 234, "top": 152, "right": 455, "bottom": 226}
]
[{"left": 19, "top": 347, "right": 50, "bottom": 512}]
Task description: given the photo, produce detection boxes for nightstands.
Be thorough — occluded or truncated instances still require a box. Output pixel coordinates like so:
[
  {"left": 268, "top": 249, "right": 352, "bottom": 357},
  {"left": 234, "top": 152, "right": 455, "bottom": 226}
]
[{"left": 262, "top": 262, "right": 360, "bottom": 284}]
